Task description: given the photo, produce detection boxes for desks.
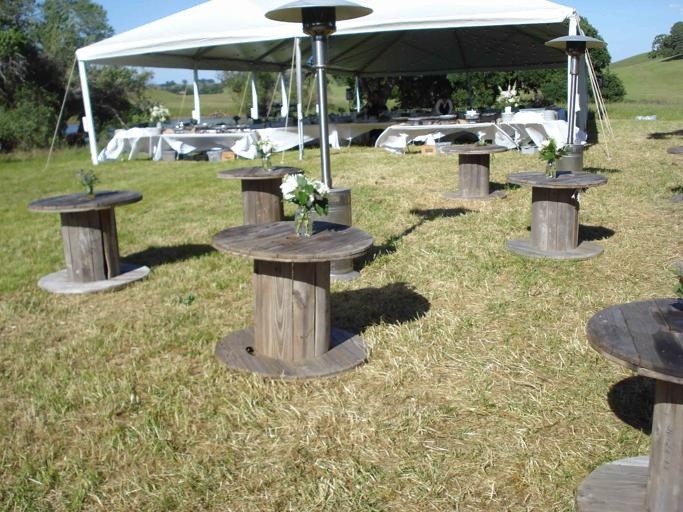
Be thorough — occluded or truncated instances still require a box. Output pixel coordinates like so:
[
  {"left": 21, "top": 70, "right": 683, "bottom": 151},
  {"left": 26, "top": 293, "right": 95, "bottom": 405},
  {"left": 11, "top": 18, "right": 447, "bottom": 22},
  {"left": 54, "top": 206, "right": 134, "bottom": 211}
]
[
  {"left": 574, "top": 298, "right": 682, "bottom": 511},
  {"left": 437, "top": 145, "right": 607, "bottom": 260},
  {"left": 26, "top": 188, "right": 152, "bottom": 296},
  {"left": 218, "top": 168, "right": 306, "bottom": 224},
  {"left": 211, "top": 221, "right": 373, "bottom": 377}
]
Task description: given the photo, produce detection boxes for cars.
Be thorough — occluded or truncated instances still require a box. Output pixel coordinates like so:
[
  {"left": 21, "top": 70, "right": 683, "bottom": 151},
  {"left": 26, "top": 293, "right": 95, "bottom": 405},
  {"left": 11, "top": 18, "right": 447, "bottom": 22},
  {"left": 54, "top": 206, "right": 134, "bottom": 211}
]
[{"left": 59, "top": 124, "right": 99, "bottom": 146}]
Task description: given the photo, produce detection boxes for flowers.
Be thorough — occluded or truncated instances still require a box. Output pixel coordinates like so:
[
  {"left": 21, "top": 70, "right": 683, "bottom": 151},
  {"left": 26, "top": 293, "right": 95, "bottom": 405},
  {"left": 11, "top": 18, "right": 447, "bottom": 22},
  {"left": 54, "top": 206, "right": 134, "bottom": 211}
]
[
  {"left": 536, "top": 138, "right": 570, "bottom": 176},
  {"left": 494, "top": 80, "right": 522, "bottom": 109},
  {"left": 250, "top": 137, "right": 279, "bottom": 171},
  {"left": 148, "top": 105, "right": 170, "bottom": 123},
  {"left": 474, "top": 131, "right": 488, "bottom": 146}
]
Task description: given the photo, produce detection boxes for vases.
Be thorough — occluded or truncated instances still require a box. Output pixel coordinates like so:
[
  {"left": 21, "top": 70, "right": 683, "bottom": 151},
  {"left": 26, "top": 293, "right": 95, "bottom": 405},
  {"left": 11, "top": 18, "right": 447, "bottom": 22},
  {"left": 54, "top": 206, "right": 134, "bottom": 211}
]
[{"left": 504, "top": 106, "right": 511, "bottom": 114}]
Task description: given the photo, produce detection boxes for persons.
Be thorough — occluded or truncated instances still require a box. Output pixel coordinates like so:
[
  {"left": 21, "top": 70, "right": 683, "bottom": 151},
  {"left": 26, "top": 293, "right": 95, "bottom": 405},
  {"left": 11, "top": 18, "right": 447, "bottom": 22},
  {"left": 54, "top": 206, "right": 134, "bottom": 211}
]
[
  {"left": 366, "top": 97, "right": 391, "bottom": 146},
  {"left": 434, "top": 94, "right": 453, "bottom": 115}
]
